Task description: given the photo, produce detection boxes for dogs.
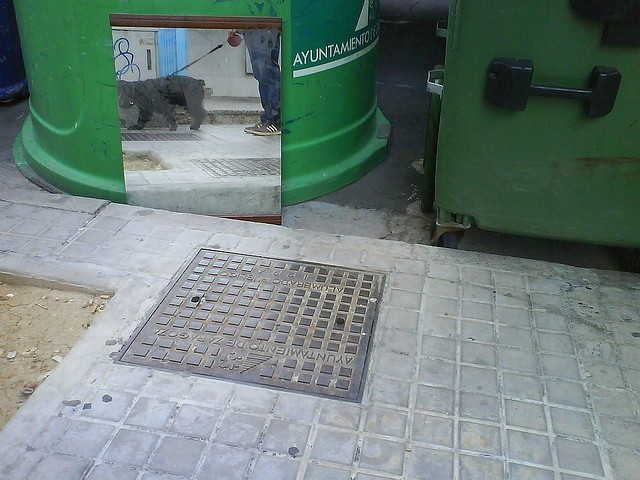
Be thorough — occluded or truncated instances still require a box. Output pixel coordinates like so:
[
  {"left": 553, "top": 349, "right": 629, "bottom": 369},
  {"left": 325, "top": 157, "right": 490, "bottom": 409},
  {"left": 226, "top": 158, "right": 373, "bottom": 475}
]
[{"left": 117, "top": 74, "right": 208, "bottom": 133}]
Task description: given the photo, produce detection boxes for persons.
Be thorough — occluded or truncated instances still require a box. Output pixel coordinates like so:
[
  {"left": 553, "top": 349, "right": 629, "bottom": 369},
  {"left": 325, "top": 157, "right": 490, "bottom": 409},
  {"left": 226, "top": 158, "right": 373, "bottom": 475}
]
[{"left": 231, "top": 25, "right": 282, "bottom": 137}]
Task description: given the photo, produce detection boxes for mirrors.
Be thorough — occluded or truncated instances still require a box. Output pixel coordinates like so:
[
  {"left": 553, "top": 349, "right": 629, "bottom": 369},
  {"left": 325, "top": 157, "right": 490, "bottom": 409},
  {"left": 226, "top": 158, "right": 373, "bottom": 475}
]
[{"left": 109, "top": 14, "right": 284, "bottom": 227}]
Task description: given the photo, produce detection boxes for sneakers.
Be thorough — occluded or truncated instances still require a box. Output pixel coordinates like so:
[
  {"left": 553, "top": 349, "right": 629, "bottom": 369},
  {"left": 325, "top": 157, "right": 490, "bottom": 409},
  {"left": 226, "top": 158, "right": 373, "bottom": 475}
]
[
  {"left": 251, "top": 119, "right": 281, "bottom": 136},
  {"left": 245, "top": 122, "right": 263, "bottom": 134}
]
